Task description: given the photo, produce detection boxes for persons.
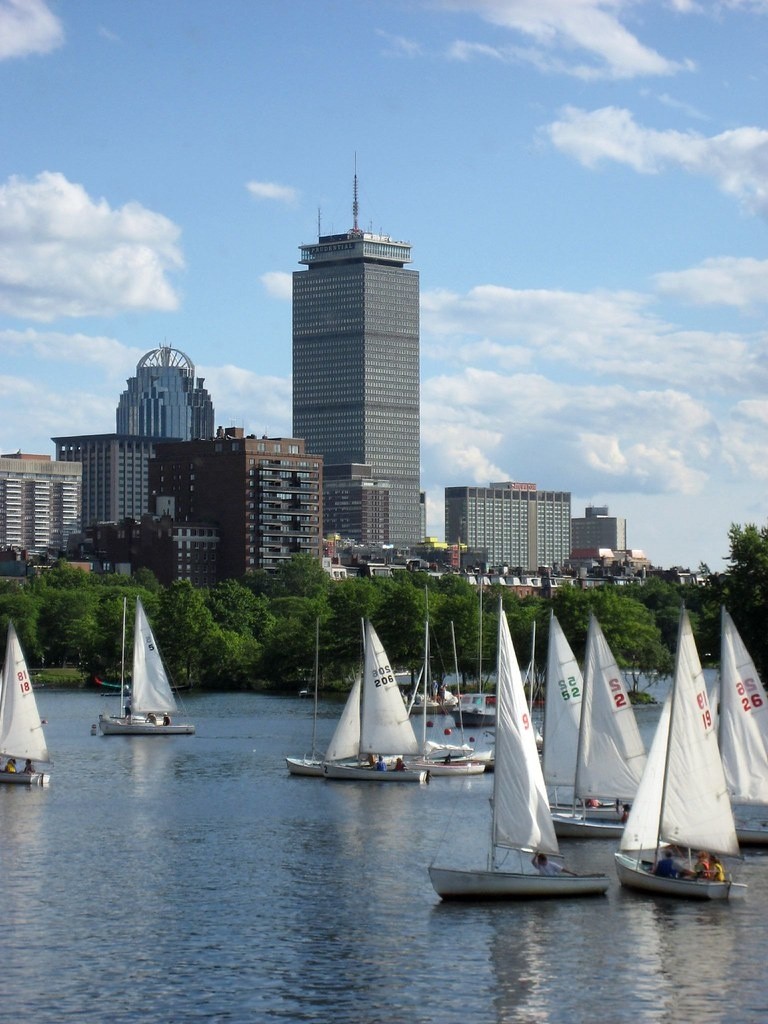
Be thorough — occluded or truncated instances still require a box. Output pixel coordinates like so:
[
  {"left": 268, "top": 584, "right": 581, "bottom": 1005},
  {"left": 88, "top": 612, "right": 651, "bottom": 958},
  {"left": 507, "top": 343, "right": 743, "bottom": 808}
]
[
  {"left": 532, "top": 851, "right": 578, "bottom": 876},
  {"left": 432, "top": 671, "right": 448, "bottom": 698},
  {"left": 655, "top": 849, "right": 695, "bottom": 878},
  {"left": 5, "top": 758, "right": 16, "bottom": 772},
  {"left": 620, "top": 804, "right": 630, "bottom": 823},
  {"left": 586, "top": 799, "right": 599, "bottom": 807},
  {"left": 366, "top": 754, "right": 377, "bottom": 765},
  {"left": 24, "top": 759, "right": 35, "bottom": 773},
  {"left": 125, "top": 697, "right": 131, "bottom": 720},
  {"left": 395, "top": 758, "right": 406, "bottom": 770},
  {"left": 163, "top": 712, "right": 170, "bottom": 725},
  {"left": 146, "top": 713, "right": 156, "bottom": 724},
  {"left": 376, "top": 755, "right": 387, "bottom": 771},
  {"left": 401, "top": 689, "right": 421, "bottom": 705},
  {"left": 695, "top": 851, "right": 726, "bottom": 880}
]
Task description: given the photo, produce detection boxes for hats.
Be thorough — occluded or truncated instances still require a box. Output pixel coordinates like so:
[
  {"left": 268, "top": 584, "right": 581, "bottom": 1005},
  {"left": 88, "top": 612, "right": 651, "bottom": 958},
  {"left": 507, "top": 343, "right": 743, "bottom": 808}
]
[
  {"left": 379, "top": 755, "right": 383, "bottom": 759},
  {"left": 397, "top": 757, "right": 402, "bottom": 760},
  {"left": 665, "top": 849, "right": 673, "bottom": 854},
  {"left": 696, "top": 851, "right": 707, "bottom": 857},
  {"left": 710, "top": 855, "right": 717, "bottom": 860}
]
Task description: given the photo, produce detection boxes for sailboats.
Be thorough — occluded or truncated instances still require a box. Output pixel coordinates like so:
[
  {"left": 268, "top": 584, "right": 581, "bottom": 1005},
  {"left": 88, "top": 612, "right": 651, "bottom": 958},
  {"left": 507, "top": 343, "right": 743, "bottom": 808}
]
[
  {"left": 426, "top": 593, "right": 613, "bottom": 901},
  {"left": 0, "top": 618, "right": 56, "bottom": 786},
  {"left": 705, "top": 605, "right": 767, "bottom": 845},
  {"left": 98, "top": 595, "right": 198, "bottom": 738},
  {"left": 489, "top": 608, "right": 648, "bottom": 839},
  {"left": 614, "top": 596, "right": 745, "bottom": 901},
  {"left": 284, "top": 615, "right": 495, "bottom": 783},
  {"left": 521, "top": 620, "right": 546, "bottom": 753}
]
